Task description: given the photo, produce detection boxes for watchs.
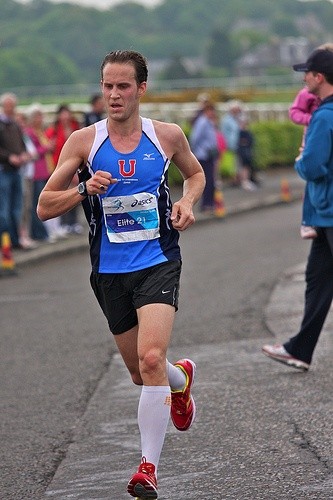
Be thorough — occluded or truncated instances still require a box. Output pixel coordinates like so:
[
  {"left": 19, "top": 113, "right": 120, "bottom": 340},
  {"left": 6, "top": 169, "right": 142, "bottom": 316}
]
[{"left": 77, "top": 180, "right": 88, "bottom": 197}]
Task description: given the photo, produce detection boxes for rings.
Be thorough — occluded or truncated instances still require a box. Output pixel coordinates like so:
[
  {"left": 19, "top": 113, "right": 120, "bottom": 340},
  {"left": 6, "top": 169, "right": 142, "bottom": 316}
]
[{"left": 100, "top": 185, "right": 105, "bottom": 191}]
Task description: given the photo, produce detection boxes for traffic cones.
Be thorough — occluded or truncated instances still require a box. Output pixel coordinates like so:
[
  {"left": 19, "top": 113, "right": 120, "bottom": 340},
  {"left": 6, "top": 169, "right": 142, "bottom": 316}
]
[
  {"left": 279, "top": 177, "right": 292, "bottom": 204},
  {"left": 0, "top": 230, "right": 19, "bottom": 278},
  {"left": 211, "top": 181, "right": 228, "bottom": 219}
]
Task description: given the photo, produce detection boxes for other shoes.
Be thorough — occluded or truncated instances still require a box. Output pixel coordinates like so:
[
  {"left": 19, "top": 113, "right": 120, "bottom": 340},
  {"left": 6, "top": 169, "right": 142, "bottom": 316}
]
[
  {"left": 17, "top": 223, "right": 83, "bottom": 251},
  {"left": 262, "top": 345, "right": 309, "bottom": 371}
]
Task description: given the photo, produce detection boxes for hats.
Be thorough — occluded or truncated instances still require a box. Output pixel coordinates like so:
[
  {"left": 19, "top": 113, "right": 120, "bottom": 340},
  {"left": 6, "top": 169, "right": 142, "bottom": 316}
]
[{"left": 293, "top": 49, "right": 333, "bottom": 72}]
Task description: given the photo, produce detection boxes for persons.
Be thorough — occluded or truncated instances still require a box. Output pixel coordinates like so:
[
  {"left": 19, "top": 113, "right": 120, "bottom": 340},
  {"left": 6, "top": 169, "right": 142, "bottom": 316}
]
[
  {"left": 262, "top": 42, "right": 333, "bottom": 371},
  {"left": 189, "top": 92, "right": 260, "bottom": 213},
  {"left": 0, "top": 50, "right": 207, "bottom": 500}
]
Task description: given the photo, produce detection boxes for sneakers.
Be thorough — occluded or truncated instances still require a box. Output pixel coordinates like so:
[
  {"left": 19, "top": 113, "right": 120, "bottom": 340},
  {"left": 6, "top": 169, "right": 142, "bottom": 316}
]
[
  {"left": 127, "top": 456, "right": 158, "bottom": 500},
  {"left": 171, "top": 359, "right": 196, "bottom": 432}
]
